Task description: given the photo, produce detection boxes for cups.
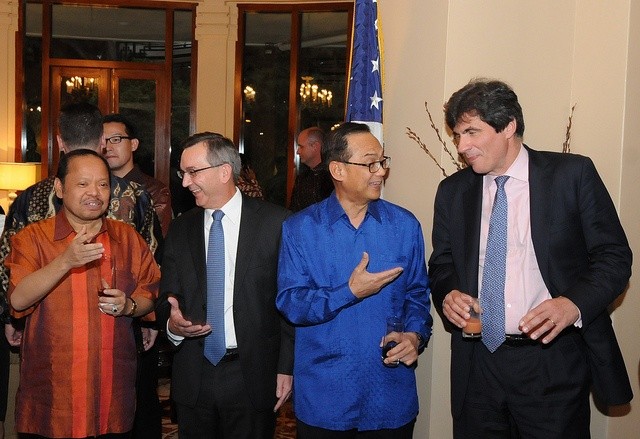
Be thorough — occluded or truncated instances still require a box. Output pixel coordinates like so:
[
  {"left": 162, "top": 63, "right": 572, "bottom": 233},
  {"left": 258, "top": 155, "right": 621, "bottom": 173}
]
[
  {"left": 461, "top": 297, "right": 483, "bottom": 338},
  {"left": 97, "top": 254, "right": 116, "bottom": 297},
  {"left": 381, "top": 315, "right": 404, "bottom": 368}
]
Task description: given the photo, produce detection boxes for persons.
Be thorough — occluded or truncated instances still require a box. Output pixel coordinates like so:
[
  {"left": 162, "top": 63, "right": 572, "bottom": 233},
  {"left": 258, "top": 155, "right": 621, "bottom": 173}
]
[
  {"left": 428, "top": 79, "right": 633, "bottom": 439},
  {"left": 274, "top": 122, "right": 433, "bottom": 438},
  {"left": 288, "top": 126, "right": 334, "bottom": 213},
  {"left": 4, "top": 148, "right": 162, "bottom": 438},
  {"left": 154, "top": 131, "right": 295, "bottom": 439},
  {"left": 102, "top": 114, "right": 171, "bottom": 239},
  {"left": 0, "top": 102, "right": 164, "bottom": 438}
]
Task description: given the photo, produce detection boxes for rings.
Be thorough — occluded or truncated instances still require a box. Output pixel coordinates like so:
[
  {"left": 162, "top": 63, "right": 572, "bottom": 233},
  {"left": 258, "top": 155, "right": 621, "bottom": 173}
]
[
  {"left": 112, "top": 305, "right": 117, "bottom": 316},
  {"left": 549, "top": 318, "right": 556, "bottom": 326}
]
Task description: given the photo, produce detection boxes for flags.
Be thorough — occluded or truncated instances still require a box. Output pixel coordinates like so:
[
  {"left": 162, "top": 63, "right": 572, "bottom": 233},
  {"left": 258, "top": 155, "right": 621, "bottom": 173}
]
[{"left": 345, "top": 0, "right": 384, "bottom": 199}]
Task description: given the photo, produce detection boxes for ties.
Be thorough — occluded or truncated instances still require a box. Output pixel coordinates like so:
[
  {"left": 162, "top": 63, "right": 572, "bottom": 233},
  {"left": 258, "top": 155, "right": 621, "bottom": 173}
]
[
  {"left": 479, "top": 175, "right": 510, "bottom": 354},
  {"left": 204, "top": 211, "right": 227, "bottom": 368}
]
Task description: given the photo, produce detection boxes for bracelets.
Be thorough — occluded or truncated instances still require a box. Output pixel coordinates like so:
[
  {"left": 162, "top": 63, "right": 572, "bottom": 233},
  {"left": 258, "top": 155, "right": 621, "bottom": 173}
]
[{"left": 126, "top": 297, "right": 139, "bottom": 318}]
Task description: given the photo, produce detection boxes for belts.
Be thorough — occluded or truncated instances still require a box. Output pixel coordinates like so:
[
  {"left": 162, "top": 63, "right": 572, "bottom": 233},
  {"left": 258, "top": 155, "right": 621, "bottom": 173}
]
[
  {"left": 220, "top": 351, "right": 241, "bottom": 365},
  {"left": 504, "top": 325, "right": 573, "bottom": 347}
]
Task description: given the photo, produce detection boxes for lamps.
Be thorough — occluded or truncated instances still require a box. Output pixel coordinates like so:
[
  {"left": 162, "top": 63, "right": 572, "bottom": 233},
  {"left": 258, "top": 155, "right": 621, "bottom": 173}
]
[
  {"left": 0, "top": 161, "right": 42, "bottom": 207},
  {"left": 300, "top": 76, "right": 318, "bottom": 102},
  {"left": 244, "top": 85, "right": 256, "bottom": 101},
  {"left": 318, "top": 89, "right": 333, "bottom": 108},
  {"left": 66, "top": 76, "right": 96, "bottom": 100}
]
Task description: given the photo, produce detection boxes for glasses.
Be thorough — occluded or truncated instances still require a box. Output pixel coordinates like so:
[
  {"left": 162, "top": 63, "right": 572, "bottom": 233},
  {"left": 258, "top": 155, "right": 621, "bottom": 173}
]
[
  {"left": 332, "top": 155, "right": 392, "bottom": 172},
  {"left": 176, "top": 164, "right": 223, "bottom": 179},
  {"left": 105, "top": 137, "right": 133, "bottom": 144}
]
